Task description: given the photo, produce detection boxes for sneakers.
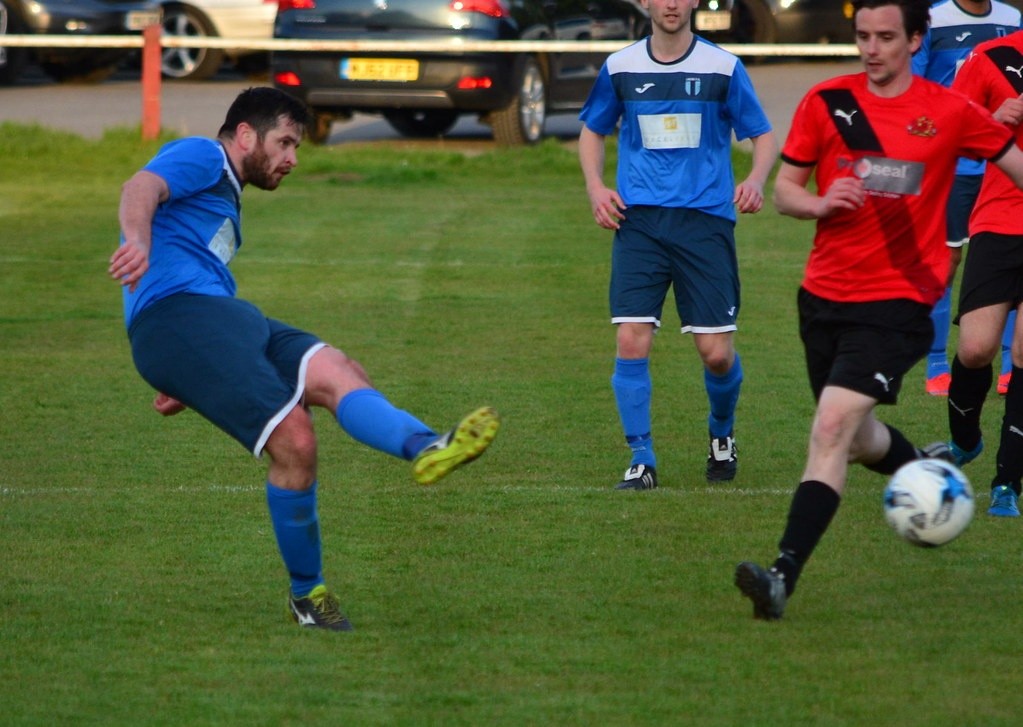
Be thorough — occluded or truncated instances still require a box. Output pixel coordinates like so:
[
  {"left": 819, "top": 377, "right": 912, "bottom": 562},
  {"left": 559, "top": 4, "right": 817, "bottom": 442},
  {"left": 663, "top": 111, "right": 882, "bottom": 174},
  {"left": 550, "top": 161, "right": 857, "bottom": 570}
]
[
  {"left": 917, "top": 441, "right": 954, "bottom": 465},
  {"left": 409, "top": 403, "right": 502, "bottom": 487},
  {"left": 287, "top": 585, "right": 350, "bottom": 633},
  {"left": 987, "top": 484, "right": 1019, "bottom": 519},
  {"left": 706, "top": 429, "right": 739, "bottom": 484},
  {"left": 923, "top": 373, "right": 953, "bottom": 396},
  {"left": 612, "top": 465, "right": 657, "bottom": 491},
  {"left": 944, "top": 433, "right": 983, "bottom": 470},
  {"left": 734, "top": 561, "right": 787, "bottom": 622},
  {"left": 997, "top": 370, "right": 1012, "bottom": 395}
]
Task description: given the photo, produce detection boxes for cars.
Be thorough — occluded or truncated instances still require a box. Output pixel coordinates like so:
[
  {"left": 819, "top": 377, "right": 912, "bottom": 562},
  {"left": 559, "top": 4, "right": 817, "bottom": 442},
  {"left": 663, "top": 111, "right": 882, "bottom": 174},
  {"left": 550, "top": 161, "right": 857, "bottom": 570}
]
[
  {"left": 1, "top": 0, "right": 168, "bottom": 86},
  {"left": 153, "top": 0, "right": 279, "bottom": 82},
  {"left": 274, "top": 0, "right": 860, "bottom": 148}
]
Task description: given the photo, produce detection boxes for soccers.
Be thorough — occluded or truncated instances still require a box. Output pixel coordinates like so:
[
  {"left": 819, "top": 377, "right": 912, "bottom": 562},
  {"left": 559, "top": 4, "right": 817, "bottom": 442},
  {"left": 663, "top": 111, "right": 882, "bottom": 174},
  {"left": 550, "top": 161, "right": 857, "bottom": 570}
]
[{"left": 883, "top": 458, "right": 976, "bottom": 547}]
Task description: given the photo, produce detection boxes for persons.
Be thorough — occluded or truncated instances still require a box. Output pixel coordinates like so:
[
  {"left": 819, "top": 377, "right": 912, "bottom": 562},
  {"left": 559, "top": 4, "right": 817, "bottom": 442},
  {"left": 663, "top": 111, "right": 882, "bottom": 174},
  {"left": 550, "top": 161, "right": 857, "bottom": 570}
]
[
  {"left": 945, "top": 29, "right": 1023, "bottom": 518},
  {"left": 910, "top": 0, "right": 1023, "bottom": 394},
  {"left": 578, "top": 1, "right": 779, "bottom": 491},
  {"left": 733, "top": 0, "right": 1023, "bottom": 620},
  {"left": 108, "top": 87, "right": 502, "bottom": 633}
]
